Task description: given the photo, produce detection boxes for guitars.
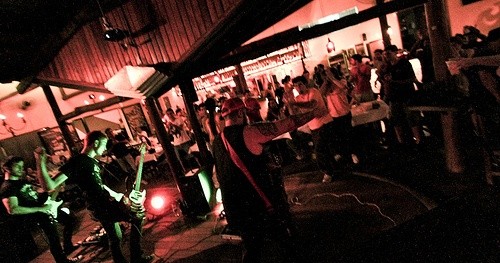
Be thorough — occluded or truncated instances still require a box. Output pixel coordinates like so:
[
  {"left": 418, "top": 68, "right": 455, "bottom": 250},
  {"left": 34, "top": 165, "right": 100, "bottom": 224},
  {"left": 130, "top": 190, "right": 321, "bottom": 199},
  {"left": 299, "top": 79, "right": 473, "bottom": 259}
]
[
  {"left": 128, "top": 142, "right": 147, "bottom": 221},
  {"left": 44, "top": 184, "right": 64, "bottom": 219}
]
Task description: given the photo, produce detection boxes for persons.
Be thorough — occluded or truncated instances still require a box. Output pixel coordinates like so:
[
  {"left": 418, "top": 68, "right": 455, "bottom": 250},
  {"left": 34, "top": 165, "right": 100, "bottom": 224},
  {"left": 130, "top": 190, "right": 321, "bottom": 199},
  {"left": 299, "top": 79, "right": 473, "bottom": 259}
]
[{"left": 0, "top": 24, "right": 500, "bottom": 263}]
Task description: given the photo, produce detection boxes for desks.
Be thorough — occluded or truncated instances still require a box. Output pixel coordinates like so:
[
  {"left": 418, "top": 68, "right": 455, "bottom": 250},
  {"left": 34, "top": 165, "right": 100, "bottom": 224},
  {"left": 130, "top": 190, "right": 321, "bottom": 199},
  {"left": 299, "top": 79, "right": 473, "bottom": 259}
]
[
  {"left": 351, "top": 100, "right": 391, "bottom": 156},
  {"left": 135, "top": 146, "right": 167, "bottom": 184}
]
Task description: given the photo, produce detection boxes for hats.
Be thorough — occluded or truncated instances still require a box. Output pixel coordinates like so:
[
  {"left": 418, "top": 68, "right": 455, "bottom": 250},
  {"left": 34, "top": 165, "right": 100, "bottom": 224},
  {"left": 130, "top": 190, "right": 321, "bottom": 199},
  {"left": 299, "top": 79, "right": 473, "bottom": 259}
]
[{"left": 221, "top": 97, "right": 252, "bottom": 117}]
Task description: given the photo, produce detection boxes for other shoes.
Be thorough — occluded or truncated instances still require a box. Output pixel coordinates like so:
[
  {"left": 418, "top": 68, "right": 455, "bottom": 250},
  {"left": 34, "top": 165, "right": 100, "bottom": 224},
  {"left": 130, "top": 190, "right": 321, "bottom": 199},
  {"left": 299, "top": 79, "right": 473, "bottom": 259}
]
[
  {"left": 141, "top": 254, "right": 156, "bottom": 263},
  {"left": 67, "top": 255, "right": 82, "bottom": 263},
  {"left": 74, "top": 241, "right": 82, "bottom": 249}
]
